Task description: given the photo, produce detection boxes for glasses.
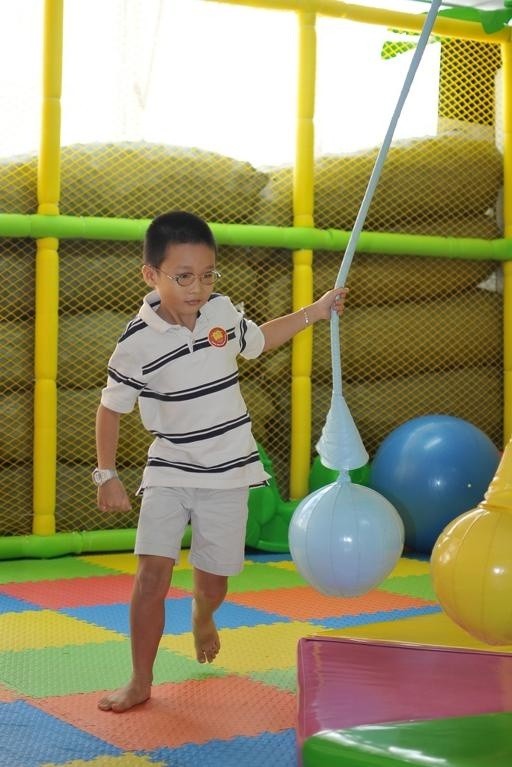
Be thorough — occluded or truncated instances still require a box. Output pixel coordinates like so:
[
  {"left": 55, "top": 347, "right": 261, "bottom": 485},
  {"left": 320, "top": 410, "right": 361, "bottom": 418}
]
[{"left": 150, "top": 264, "right": 220, "bottom": 287}]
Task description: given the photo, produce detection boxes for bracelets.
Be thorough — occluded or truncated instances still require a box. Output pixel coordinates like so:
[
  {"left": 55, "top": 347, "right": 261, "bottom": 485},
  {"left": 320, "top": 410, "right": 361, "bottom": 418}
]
[{"left": 301, "top": 308, "right": 309, "bottom": 328}]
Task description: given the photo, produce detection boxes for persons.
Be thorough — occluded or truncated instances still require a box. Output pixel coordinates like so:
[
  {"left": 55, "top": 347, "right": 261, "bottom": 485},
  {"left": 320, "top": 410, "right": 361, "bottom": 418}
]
[{"left": 87, "top": 208, "right": 352, "bottom": 717}]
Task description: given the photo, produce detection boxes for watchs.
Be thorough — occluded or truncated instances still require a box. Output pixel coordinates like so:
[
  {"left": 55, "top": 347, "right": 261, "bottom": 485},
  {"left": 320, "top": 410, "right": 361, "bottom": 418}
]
[{"left": 90, "top": 465, "right": 119, "bottom": 488}]
